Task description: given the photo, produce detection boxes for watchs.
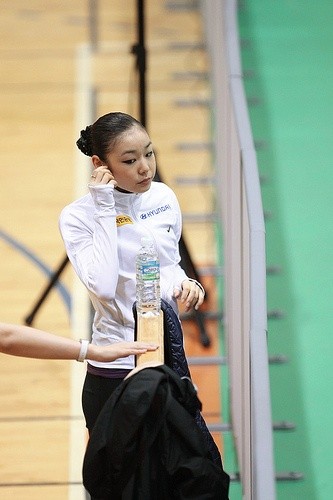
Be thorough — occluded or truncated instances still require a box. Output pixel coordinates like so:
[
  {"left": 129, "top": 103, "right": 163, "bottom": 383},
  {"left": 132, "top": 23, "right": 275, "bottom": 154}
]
[{"left": 77, "top": 337, "right": 90, "bottom": 363}]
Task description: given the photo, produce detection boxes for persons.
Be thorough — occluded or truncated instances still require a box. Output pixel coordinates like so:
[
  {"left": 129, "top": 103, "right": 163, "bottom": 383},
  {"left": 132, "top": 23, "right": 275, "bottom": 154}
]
[
  {"left": 58, "top": 111, "right": 204, "bottom": 437},
  {"left": 0, "top": 320, "right": 159, "bottom": 363}
]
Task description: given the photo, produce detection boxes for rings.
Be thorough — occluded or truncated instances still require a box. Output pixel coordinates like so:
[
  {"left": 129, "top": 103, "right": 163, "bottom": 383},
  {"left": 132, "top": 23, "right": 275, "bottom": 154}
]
[{"left": 90, "top": 175, "right": 97, "bottom": 179}]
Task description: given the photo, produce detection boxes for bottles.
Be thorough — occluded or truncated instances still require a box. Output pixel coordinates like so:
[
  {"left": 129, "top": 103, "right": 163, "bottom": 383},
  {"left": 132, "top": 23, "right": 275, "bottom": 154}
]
[{"left": 136, "top": 238, "right": 162, "bottom": 317}]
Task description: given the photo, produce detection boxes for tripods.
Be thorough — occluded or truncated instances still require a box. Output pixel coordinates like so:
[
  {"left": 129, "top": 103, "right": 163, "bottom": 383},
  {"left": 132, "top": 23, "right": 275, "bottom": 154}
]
[{"left": 26, "top": 0, "right": 211, "bottom": 347}]
